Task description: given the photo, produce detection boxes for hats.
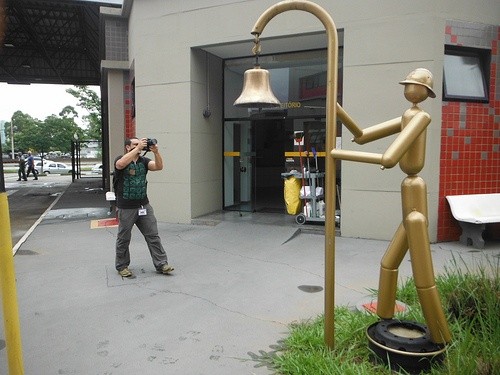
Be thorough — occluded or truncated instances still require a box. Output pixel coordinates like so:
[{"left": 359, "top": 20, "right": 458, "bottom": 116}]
[{"left": 27, "top": 151, "right": 32, "bottom": 154}]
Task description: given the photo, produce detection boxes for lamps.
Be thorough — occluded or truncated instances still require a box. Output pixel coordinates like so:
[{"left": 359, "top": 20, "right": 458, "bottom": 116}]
[{"left": 365, "top": 319, "right": 446, "bottom": 374}]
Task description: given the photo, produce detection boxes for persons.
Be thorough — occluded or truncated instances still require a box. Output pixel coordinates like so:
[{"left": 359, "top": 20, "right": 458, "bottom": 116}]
[
  {"left": 26, "top": 151, "right": 39, "bottom": 180},
  {"left": 329, "top": 68, "right": 452, "bottom": 344},
  {"left": 114, "top": 137, "right": 175, "bottom": 277},
  {"left": 17, "top": 152, "right": 28, "bottom": 181}
]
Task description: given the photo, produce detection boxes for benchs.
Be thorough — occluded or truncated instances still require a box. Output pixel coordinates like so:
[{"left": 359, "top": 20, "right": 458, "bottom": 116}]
[{"left": 446, "top": 193, "right": 500, "bottom": 248}]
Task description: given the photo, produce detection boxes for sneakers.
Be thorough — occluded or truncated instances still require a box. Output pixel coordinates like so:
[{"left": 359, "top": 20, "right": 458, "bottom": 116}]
[
  {"left": 156, "top": 264, "right": 175, "bottom": 274},
  {"left": 119, "top": 266, "right": 132, "bottom": 277}
]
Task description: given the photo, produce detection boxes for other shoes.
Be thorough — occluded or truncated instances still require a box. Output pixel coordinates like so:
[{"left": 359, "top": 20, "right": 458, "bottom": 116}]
[
  {"left": 16, "top": 178, "right": 21, "bottom": 181},
  {"left": 24, "top": 178, "right": 27, "bottom": 181},
  {"left": 33, "top": 178, "right": 38, "bottom": 180}
]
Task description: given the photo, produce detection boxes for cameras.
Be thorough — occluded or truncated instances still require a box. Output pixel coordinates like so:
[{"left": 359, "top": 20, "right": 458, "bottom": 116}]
[{"left": 142, "top": 139, "right": 158, "bottom": 151}]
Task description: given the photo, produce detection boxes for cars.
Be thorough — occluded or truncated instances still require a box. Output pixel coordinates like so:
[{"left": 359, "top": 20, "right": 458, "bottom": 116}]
[
  {"left": 9, "top": 150, "right": 82, "bottom": 176},
  {"left": 90, "top": 163, "right": 103, "bottom": 174}
]
